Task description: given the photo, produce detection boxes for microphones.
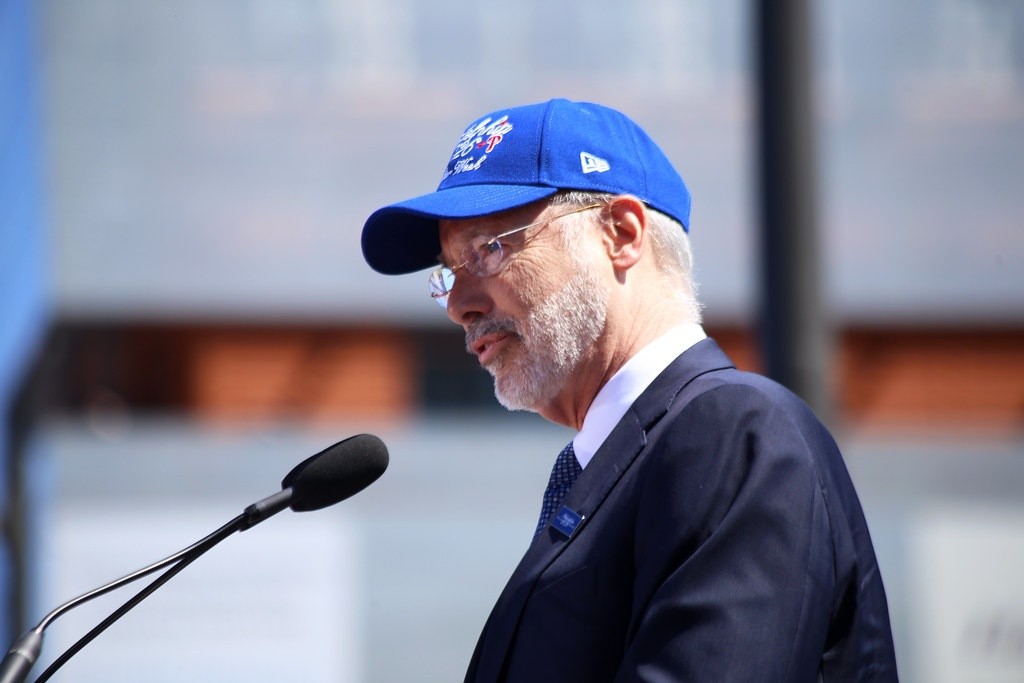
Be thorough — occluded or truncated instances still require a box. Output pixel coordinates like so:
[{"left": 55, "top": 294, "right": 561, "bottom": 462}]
[{"left": 3, "top": 433, "right": 388, "bottom": 682}]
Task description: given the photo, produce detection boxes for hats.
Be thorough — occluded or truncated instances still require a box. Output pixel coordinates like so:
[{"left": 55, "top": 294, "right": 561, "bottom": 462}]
[{"left": 360, "top": 98, "right": 691, "bottom": 276}]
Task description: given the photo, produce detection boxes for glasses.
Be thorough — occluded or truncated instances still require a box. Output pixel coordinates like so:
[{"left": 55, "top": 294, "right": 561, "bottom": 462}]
[{"left": 428, "top": 204, "right": 610, "bottom": 309}]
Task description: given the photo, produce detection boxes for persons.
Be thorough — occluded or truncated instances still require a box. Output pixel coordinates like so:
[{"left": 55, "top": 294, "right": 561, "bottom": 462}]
[{"left": 430, "top": 98, "right": 901, "bottom": 682}]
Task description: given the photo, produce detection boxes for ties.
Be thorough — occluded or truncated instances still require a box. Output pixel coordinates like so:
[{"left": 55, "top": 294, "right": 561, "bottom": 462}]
[{"left": 531, "top": 441, "right": 582, "bottom": 543}]
